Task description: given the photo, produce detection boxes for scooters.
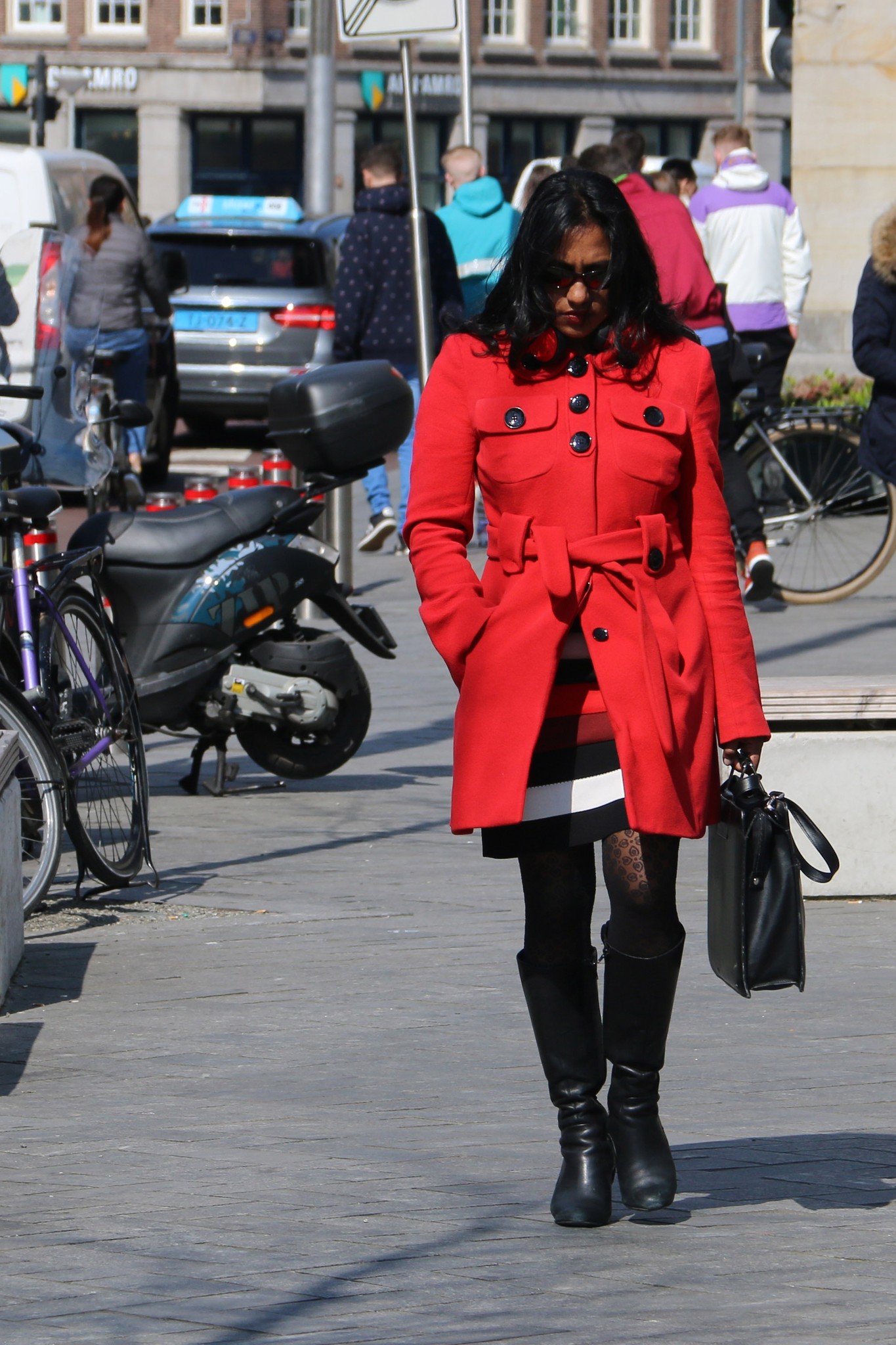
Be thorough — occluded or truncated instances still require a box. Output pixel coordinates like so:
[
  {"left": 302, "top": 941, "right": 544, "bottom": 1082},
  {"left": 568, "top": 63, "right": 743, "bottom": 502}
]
[{"left": 0, "top": 356, "right": 414, "bottom": 800}]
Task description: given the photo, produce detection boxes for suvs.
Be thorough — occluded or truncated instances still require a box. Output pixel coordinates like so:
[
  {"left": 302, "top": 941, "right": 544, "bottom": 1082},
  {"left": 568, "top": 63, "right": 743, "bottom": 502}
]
[{"left": 148, "top": 193, "right": 356, "bottom": 440}]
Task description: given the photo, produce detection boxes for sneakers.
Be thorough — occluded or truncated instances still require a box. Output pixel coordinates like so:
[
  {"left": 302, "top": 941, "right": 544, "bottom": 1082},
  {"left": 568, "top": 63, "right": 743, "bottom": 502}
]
[
  {"left": 358, "top": 507, "right": 396, "bottom": 552},
  {"left": 744, "top": 540, "right": 773, "bottom": 601},
  {"left": 394, "top": 542, "right": 411, "bottom": 556}
]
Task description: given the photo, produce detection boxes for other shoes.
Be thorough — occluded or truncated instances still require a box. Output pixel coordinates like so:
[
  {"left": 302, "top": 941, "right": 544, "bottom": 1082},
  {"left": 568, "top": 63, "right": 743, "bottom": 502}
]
[{"left": 123, "top": 472, "right": 145, "bottom": 506}]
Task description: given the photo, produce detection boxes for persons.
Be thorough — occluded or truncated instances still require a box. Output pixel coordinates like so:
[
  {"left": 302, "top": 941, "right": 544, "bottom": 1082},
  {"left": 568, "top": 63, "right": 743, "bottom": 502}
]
[
  {"left": 402, "top": 168, "right": 770, "bottom": 1227},
  {"left": 332, "top": 143, "right": 464, "bottom": 552},
  {"left": 522, "top": 127, "right": 811, "bottom": 602},
  {"left": 64, "top": 173, "right": 173, "bottom": 473},
  {"left": 852, "top": 201, "right": 896, "bottom": 487},
  {"left": 0, "top": 260, "right": 21, "bottom": 382},
  {"left": 433, "top": 145, "right": 524, "bottom": 322}
]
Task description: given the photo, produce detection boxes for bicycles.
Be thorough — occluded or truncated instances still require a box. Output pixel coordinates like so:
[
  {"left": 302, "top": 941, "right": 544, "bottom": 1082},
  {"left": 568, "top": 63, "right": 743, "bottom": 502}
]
[
  {"left": 723, "top": 338, "right": 896, "bottom": 606},
  {"left": 1, "top": 380, "right": 161, "bottom": 922}
]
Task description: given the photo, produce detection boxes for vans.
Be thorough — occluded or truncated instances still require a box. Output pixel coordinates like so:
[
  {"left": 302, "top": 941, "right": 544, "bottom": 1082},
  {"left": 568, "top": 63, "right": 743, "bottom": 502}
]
[{"left": 0, "top": 141, "right": 148, "bottom": 427}]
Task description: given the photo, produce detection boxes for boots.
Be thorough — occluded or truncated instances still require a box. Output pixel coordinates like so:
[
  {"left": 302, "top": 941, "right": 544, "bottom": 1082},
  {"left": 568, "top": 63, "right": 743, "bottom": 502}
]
[
  {"left": 597, "top": 920, "right": 686, "bottom": 1212},
  {"left": 516, "top": 944, "right": 616, "bottom": 1227}
]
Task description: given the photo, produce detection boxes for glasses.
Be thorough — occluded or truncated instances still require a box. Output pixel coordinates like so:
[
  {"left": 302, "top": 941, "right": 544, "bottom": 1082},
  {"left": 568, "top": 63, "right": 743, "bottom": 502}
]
[{"left": 547, "top": 265, "right": 611, "bottom": 291}]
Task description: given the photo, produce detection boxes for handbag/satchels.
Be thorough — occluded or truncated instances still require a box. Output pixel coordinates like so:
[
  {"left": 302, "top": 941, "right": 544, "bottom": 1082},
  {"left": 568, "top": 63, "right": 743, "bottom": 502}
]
[{"left": 706, "top": 754, "right": 840, "bottom": 999}]
